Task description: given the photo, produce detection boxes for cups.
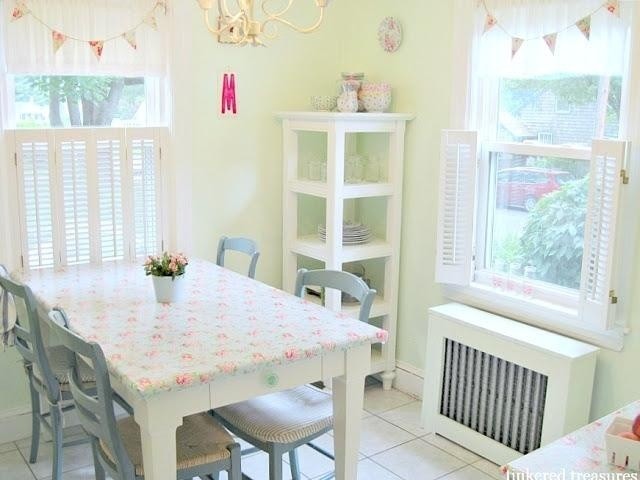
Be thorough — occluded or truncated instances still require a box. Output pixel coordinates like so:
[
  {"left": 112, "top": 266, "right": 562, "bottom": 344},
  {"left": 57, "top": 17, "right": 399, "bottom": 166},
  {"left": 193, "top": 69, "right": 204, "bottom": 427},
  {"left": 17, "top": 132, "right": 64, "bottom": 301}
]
[{"left": 307, "top": 154, "right": 381, "bottom": 185}]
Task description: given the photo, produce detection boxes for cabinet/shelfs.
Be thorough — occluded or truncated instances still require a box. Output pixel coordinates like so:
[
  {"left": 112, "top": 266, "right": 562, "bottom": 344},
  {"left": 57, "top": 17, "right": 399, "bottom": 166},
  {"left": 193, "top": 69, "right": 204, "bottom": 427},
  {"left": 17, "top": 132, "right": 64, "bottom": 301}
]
[{"left": 275, "top": 110, "right": 415, "bottom": 392}]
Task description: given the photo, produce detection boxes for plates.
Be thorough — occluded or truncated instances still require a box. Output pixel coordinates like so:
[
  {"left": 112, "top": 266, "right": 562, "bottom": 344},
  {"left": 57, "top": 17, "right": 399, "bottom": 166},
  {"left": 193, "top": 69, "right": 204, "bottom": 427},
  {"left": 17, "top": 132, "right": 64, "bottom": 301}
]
[
  {"left": 375, "top": 15, "right": 404, "bottom": 54},
  {"left": 318, "top": 220, "right": 373, "bottom": 245}
]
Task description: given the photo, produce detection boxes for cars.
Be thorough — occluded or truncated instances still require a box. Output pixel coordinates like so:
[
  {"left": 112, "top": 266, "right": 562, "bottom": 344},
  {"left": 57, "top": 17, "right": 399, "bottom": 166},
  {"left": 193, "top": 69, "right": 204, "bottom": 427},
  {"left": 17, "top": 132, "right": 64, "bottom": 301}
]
[{"left": 498, "top": 167, "right": 573, "bottom": 212}]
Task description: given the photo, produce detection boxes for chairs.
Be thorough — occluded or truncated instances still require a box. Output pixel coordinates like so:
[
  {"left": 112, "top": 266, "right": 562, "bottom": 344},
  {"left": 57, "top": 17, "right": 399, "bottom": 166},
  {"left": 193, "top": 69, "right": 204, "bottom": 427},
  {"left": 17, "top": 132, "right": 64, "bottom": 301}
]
[{"left": 0, "top": 234, "right": 376, "bottom": 480}]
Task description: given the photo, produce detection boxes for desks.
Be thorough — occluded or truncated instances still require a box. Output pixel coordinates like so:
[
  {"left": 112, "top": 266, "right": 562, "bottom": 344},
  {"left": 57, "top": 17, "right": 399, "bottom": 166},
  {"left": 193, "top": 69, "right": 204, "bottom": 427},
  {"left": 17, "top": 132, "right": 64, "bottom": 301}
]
[
  {"left": 18, "top": 256, "right": 389, "bottom": 480},
  {"left": 496, "top": 394, "right": 640, "bottom": 480}
]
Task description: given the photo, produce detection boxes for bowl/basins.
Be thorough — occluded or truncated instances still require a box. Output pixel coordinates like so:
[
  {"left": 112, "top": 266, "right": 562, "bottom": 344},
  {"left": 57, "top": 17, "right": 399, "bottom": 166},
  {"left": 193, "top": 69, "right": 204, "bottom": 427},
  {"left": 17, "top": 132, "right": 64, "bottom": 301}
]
[
  {"left": 311, "top": 96, "right": 338, "bottom": 112},
  {"left": 336, "top": 80, "right": 392, "bottom": 113}
]
